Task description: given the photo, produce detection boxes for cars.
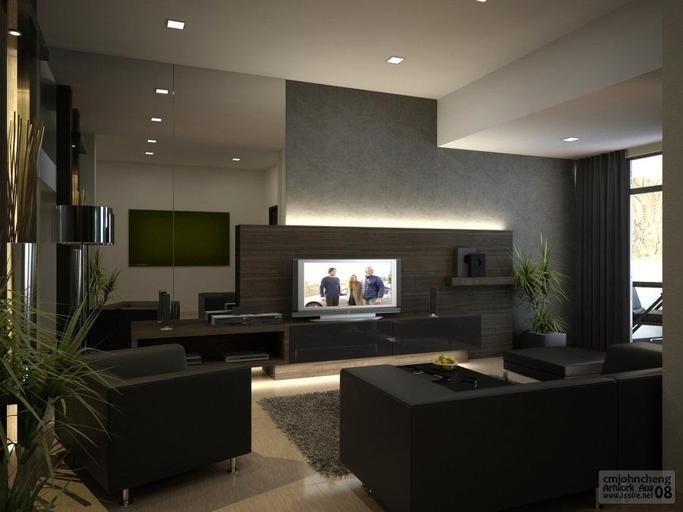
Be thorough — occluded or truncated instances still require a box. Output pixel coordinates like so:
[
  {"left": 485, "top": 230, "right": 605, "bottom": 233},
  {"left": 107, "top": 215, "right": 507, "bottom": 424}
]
[{"left": 303, "top": 283, "right": 393, "bottom": 306}]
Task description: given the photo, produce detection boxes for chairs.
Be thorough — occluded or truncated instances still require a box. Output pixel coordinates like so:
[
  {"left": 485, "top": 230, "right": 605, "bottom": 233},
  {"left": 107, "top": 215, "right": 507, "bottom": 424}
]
[{"left": 631, "top": 293, "right": 663, "bottom": 333}]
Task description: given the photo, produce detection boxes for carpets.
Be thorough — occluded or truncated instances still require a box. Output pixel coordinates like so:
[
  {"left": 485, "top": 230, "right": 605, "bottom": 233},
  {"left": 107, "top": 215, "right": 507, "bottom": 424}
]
[{"left": 256, "top": 388, "right": 356, "bottom": 480}]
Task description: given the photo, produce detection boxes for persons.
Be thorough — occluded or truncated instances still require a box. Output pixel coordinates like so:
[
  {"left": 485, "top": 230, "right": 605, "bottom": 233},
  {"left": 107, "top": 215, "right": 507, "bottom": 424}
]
[
  {"left": 345, "top": 274, "right": 363, "bottom": 305},
  {"left": 320, "top": 267, "right": 340, "bottom": 306},
  {"left": 362, "top": 265, "right": 385, "bottom": 305}
]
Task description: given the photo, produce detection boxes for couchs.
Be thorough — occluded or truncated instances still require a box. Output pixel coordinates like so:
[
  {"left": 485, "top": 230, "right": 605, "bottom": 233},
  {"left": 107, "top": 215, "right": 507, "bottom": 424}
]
[
  {"left": 339, "top": 343, "right": 662, "bottom": 512},
  {"left": 50, "top": 341, "right": 254, "bottom": 508}
]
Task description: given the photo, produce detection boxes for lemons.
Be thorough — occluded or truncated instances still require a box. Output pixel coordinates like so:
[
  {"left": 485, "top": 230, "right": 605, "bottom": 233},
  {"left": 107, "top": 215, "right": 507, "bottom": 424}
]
[{"left": 435, "top": 354, "right": 454, "bottom": 364}]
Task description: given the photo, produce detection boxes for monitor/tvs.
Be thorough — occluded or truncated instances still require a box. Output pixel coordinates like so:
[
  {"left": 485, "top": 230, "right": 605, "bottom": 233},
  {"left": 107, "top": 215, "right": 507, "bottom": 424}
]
[{"left": 290, "top": 256, "right": 402, "bottom": 324}]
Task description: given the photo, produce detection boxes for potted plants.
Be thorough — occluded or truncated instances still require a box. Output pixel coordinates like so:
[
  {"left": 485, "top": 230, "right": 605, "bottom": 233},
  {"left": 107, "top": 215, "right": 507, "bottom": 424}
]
[{"left": 511, "top": 234, "right": 570, "bottom": 348}]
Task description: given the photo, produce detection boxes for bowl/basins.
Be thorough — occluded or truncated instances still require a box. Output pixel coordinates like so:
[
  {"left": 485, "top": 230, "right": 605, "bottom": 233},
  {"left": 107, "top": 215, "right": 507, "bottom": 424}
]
[{"left": 432, "top": 360, "right": 458, "bottom": 371}]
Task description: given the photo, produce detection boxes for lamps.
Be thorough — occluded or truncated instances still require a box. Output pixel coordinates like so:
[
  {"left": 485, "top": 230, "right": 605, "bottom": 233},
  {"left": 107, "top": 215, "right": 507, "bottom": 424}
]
[{"left": 51, "top": 204, "right": 116, "bottom": 353}]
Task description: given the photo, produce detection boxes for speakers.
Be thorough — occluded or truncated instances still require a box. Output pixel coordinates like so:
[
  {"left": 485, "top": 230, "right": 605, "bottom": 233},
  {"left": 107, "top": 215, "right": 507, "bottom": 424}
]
[
  {"left": 427, "top": 287, "right": 440, "bottom": 318},
  {"left": 160, "top": 292, "right": 174, "bottom": 331},
  {"left": 156, "top": 289, "right": 167, "bottom": 323}
]
